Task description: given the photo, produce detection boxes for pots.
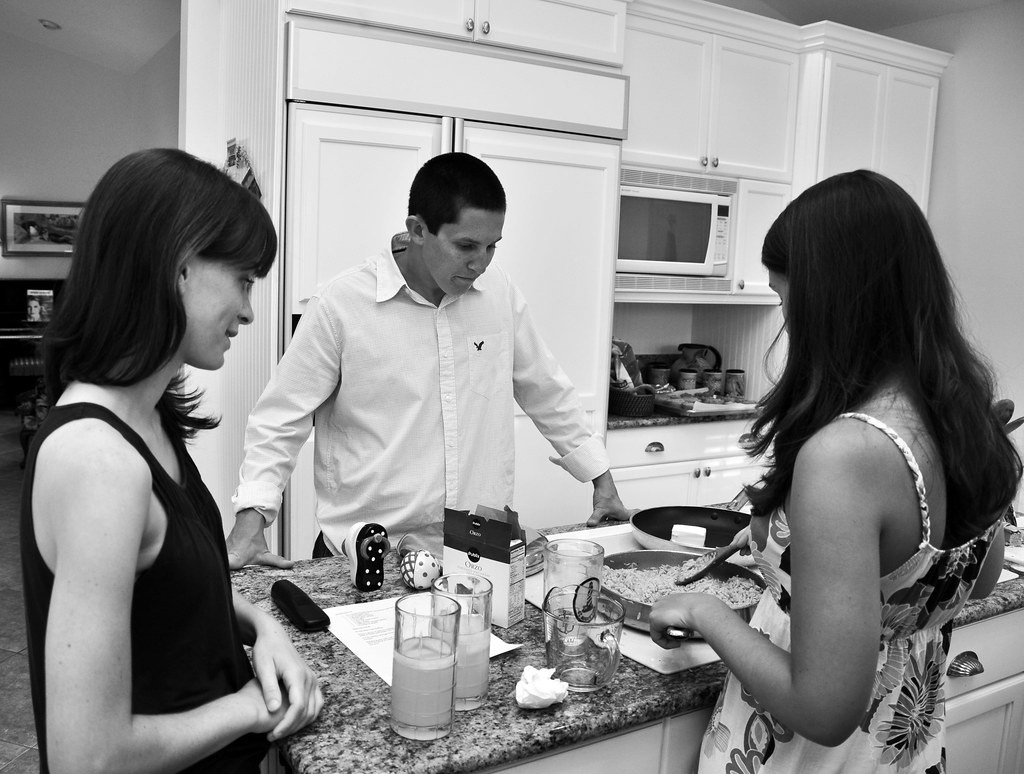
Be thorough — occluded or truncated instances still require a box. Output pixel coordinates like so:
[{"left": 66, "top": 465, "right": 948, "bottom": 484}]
[
  {"left": 628, "top": 479, "right": 767, "bottom": 567},
  {"left": 593, "top": 551, "right": 767, "bottom": 642}
]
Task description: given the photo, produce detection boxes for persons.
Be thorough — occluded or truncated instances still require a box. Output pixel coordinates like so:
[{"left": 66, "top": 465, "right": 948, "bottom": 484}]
[
  {"left": 648, "top": 169, "right": 1023, "bottom": 774},
  {"left": 21, "top": 148, "right": 327, "bottom": 774},
  {"left": 27, "top": 296, "right": 43, "bottom": 321},
  {"left": 226, "top": 152, "right": 644, "bottom": 571}
]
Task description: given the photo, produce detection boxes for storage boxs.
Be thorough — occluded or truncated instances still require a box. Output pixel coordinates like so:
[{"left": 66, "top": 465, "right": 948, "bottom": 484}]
[{"left": 443, "top": 505, "right": 527, "bottom": 629}]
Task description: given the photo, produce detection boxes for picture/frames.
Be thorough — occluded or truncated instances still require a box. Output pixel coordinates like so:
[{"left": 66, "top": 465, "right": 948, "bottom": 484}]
[{"left": 0, "top": 198, "right": 85, "bottom": 258}]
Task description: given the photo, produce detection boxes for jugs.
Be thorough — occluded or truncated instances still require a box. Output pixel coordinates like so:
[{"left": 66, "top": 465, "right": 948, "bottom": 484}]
[{"left": 668, "top": 343, "right": 722, "bottom": 388}]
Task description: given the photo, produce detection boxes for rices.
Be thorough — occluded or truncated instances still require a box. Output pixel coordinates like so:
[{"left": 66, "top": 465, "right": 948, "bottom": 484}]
[{"left": 602, "top": 553, "right": 762, "bottom": 610}]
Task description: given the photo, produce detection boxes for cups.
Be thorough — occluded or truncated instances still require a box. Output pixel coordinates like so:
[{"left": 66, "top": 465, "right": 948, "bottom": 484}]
[
  {"left": 429, "top": 573, "right": 494, "bottom": 712},
  {"left": 649, "top": 367, "right": 671, "bottom": 387},
  {"left": 677, "top": 369, "right": 697, "bottom": 397},
  {"left": 543, "top": 539, "right": 605, "bottom": 657},
  {"left": 542, "top": 592, "right": 626, "bottom": 692},
  {"left": 725, "top": 368, "right": 745, "bottom": 399},
  {"left": 389, "top": 592, "right": 461, "bottom": 741},
  {"left": 704, "top": 369, "right": 723, "bottom": 403}
]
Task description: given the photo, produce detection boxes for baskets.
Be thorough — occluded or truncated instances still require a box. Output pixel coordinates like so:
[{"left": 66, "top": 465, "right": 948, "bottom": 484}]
[{"left": 609, "top": 383, "right": 655, "bottom": 418}]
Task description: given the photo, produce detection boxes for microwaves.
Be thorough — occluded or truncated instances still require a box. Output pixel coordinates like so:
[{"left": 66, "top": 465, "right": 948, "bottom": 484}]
[{"left": 613, "top": 184, "right": 732, "bottom": 278}]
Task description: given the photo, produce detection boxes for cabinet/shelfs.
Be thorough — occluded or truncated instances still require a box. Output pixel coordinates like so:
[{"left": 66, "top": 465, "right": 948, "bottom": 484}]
[
  {"left": 177, "top": 2, "right": 954, "bottom": 564},
  {"left": 0, "top": 327, "right": 46, "bottom": 416},
  {"left": 479, "top": 608, "right": 1024, "bottom": 774}
]
